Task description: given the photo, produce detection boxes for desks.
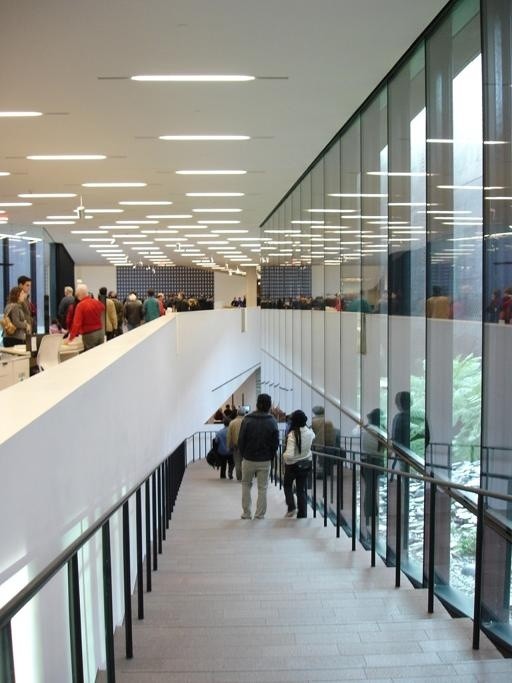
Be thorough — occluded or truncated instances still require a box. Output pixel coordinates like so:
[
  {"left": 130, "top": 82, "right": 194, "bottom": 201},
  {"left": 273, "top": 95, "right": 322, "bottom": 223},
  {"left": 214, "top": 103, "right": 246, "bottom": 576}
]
[
  {"left": 9, "top": 334, "right": 83, "bottom": 367},
  {"left": 0, "top": 347, "right": 32, "bottom": 391}
]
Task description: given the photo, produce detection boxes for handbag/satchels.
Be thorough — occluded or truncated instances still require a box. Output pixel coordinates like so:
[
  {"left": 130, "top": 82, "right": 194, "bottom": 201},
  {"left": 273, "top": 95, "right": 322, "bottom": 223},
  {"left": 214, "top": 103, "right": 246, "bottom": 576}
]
[
  {"left": 207, "top": 439, "right": 222, "bottom": 467},
  {"left": 297, "top": 460, "right": 312, "bottom": 471},
  {"left": 0, "top": 315, "right": 17, "bottom": 336}
]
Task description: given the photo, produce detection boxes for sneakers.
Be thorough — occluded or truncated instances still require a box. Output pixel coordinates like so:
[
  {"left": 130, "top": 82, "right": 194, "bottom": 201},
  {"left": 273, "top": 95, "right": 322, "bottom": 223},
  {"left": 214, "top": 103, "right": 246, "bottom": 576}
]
[{"left": 286, "top": 509, "right": 299, "bottom": 517}]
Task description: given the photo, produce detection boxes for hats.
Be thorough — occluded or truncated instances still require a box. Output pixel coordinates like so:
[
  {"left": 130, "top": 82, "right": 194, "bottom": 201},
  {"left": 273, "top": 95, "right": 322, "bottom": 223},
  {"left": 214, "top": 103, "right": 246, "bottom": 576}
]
[
  {"left": 312, "top": 405, "right": 323, "bottom": 415},
  {"left": 237, "top": 408, "right": 248, "bottom": 416}
]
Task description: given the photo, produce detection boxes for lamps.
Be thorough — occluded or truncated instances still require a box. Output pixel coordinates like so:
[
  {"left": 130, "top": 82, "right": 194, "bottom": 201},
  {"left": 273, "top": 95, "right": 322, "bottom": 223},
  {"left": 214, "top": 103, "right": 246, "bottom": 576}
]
[{"left": 77, "top": 195, "right": 85, "bottom": 222}]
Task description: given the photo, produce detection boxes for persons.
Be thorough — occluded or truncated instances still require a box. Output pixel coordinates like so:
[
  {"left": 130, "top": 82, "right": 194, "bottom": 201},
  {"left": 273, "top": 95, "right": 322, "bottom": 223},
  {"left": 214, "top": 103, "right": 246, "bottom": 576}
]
[
  {"left": 316, "top": 393, "right": 433, "bottom": 530},
  {"left": 2, "top": 274, "right": 249, "bottom": 349},
  {"left": 211, "top": 393, "right": 316, "bottom": 518},
  {"left": 256, "top": 285, "right": 512, "bottom": 324}
]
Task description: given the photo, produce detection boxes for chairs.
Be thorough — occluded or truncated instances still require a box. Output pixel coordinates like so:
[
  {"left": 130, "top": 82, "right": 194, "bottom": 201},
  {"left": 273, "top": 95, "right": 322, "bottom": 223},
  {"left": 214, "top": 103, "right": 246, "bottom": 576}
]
[{"left": 35, "top": 333, "right": 64, "bottom": 372}]
